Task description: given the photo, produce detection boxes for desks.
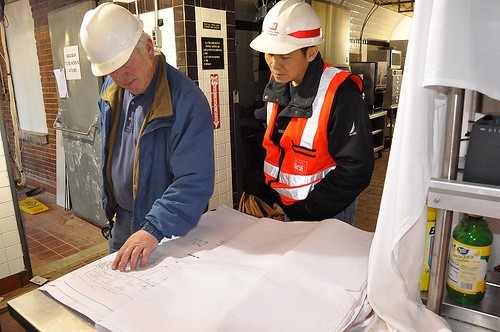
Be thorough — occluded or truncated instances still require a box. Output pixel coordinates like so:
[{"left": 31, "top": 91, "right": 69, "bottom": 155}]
[
  {"left": 7, "top": 286, "right": 100, "bottom": 332},
  {"left": 383, "top": 103, "right": 399, "bottom": 151}
]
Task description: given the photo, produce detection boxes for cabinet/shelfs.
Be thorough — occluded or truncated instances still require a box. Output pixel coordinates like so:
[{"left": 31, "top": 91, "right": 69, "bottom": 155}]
[
  {"left": 368, "top": 110, "right": 388, "bottom": 158},
  {"left": 419, "top": 87, "right": 500, "bottom": 332}
]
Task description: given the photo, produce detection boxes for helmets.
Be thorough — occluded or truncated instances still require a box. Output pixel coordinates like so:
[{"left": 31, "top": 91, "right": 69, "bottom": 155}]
[
  {"left": 80, "top": 2, "right": 143, "bottom": 76},
  {"left": 249, "top": 0, "right": 325, "bottom": 54}
]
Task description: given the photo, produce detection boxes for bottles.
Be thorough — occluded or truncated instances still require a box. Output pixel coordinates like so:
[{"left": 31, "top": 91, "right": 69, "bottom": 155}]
[
  {"left": 419, "top": 208, "right": 437, "bottom": 292},
  {"left": 444, "top": 213, "right": 491, "bottom": 306}
]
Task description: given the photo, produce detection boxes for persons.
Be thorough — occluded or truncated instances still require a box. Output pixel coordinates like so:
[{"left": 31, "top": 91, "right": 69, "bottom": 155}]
[
  {"left": 79, "top": 2, "right": 215, "bottom": 273},
  {"left": 248, "top": 0, "right": 376, "bottom": 227}
]
